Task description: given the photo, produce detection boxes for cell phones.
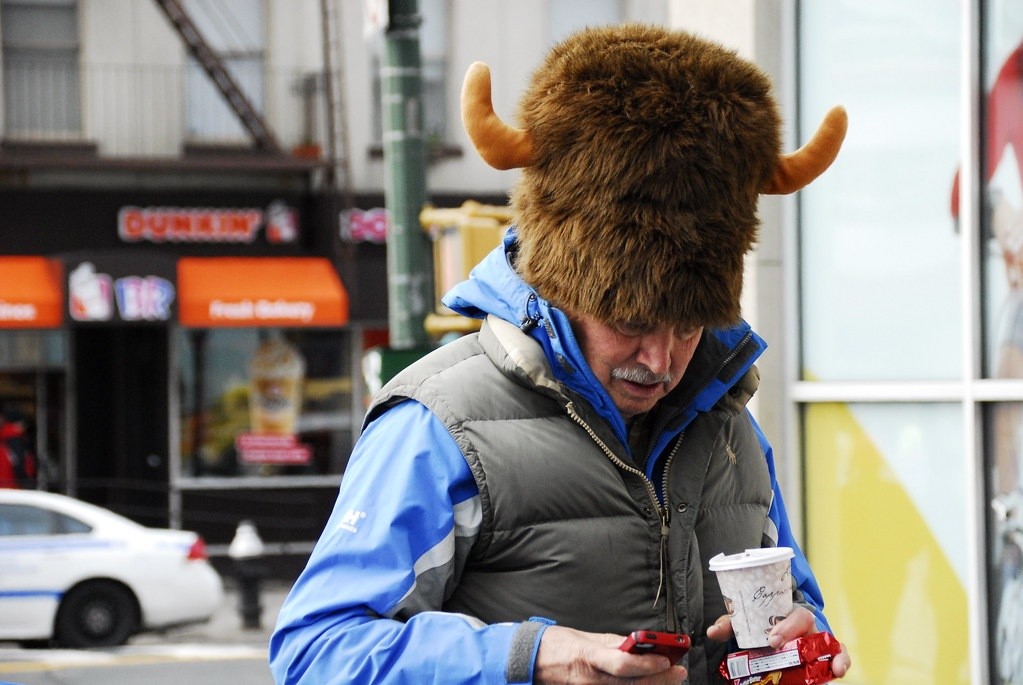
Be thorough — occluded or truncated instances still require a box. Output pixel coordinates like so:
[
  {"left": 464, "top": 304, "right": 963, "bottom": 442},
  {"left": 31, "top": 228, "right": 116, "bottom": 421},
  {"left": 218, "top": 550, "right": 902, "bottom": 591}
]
[{"left": 618, "top": 630, "right": 691, "bottom": 667}]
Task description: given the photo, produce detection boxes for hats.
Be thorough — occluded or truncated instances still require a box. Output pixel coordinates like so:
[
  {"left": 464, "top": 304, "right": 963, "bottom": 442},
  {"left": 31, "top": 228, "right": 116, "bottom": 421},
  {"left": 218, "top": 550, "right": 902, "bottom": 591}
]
[{"left": 463, "top": 23, "right": 847, "bottom": 329}]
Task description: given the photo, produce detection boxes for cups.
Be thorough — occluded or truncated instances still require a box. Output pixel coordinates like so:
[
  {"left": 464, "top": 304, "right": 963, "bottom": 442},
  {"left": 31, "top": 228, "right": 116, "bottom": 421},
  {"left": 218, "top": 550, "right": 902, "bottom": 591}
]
[
  {"left": 708, "top": 547, "right": 797, "bottom": 649},
  {"left": 239, "top": 352, "right": 306, "bottom": 440}
]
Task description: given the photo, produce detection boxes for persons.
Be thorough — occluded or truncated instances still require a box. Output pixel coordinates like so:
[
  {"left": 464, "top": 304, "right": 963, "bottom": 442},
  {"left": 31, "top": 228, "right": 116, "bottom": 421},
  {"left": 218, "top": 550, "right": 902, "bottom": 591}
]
[
  {"left": 0, "top": 404, "right": 39, "bottom": 491},
  {"left": 268, "top": 24, "right": 853, "bottom": 685}
]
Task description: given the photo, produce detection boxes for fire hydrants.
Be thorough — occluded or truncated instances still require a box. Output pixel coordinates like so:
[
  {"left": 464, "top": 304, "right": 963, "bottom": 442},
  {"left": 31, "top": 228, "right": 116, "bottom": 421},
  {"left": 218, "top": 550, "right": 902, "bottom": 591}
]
[{"left": 228, "top": 523, "right": 269, "bottom": 631}]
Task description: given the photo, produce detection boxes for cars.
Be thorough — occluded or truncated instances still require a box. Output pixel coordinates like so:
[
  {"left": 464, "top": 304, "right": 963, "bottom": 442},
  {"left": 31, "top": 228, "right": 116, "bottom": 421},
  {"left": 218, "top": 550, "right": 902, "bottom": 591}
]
[{"left": 0, "top": 488, "right": 219, "bottom": 650}]
